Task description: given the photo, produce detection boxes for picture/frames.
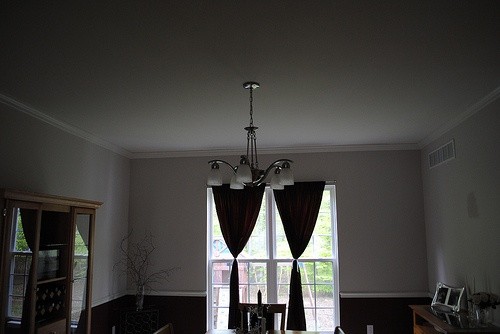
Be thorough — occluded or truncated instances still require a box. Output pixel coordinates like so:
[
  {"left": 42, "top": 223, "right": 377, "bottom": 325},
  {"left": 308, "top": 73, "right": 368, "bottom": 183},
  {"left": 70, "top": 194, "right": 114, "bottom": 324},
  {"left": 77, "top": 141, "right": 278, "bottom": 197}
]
[{"left": 430, "top": 281, "right": 465, "bottom": 312}]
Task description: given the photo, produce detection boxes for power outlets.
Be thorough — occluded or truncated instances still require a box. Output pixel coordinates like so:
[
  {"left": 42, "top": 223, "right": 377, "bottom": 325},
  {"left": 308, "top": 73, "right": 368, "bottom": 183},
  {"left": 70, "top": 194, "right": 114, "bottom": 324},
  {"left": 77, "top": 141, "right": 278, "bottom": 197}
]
[{"left": 366, "top": 325, "right": 374, "bottom": 334}]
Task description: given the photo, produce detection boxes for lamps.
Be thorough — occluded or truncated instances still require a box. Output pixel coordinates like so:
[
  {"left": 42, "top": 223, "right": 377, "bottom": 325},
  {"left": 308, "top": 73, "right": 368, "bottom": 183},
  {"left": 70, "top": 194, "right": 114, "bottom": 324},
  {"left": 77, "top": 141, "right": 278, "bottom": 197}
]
[{"left": 207, "top": 81, "right": 294, "bottom": 189}]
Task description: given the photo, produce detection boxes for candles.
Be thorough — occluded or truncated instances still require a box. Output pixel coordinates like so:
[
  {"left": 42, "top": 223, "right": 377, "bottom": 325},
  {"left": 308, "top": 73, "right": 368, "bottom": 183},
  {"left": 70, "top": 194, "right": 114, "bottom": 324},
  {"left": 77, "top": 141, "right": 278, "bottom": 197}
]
[{"left": 257, "top": 290, "right": 263, "bottom": 308}]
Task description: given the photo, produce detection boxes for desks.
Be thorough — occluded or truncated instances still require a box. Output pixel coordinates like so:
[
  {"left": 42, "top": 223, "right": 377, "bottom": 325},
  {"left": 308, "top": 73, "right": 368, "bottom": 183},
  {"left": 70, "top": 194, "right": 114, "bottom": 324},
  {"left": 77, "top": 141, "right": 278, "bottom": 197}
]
[{"left": 408, "top": 304, "right": 500, "bottom": 334}]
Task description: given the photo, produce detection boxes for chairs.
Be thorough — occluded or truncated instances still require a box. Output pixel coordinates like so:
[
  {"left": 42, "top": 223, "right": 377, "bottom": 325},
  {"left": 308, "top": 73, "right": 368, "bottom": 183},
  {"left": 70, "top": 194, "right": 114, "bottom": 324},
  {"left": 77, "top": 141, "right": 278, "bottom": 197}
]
[{"left": 236, "top": 302, "right": 287, "bottom": 330}]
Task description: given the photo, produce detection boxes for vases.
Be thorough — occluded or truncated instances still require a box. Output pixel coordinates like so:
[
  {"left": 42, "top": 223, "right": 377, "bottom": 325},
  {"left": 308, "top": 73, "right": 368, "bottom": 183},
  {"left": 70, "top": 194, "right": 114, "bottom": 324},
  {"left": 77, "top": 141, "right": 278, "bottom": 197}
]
[{"left": 478, "top": 311, "right": 489, "bottom": 327}]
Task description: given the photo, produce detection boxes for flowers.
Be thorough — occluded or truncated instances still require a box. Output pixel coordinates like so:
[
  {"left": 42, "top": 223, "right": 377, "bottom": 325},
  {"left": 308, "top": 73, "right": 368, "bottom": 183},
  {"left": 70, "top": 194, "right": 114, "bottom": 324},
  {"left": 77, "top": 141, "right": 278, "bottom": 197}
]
[{"left": 469, "top": 291, "right": 500, "bottom": 311}]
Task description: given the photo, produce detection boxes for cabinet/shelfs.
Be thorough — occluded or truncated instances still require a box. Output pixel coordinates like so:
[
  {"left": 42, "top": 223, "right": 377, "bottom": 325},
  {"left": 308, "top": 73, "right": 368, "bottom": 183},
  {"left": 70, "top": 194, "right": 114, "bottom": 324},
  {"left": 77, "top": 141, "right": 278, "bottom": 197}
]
[{"left": 0, "top": 190, "right": 103, "bottom": 334}]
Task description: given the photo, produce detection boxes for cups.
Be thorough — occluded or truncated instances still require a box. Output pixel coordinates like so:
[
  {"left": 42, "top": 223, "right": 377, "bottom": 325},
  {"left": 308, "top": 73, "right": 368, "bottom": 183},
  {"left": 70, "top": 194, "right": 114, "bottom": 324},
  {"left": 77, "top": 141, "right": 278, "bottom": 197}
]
[{"left": 459, "top": 315, "right": 476, "bottom": 329}]
